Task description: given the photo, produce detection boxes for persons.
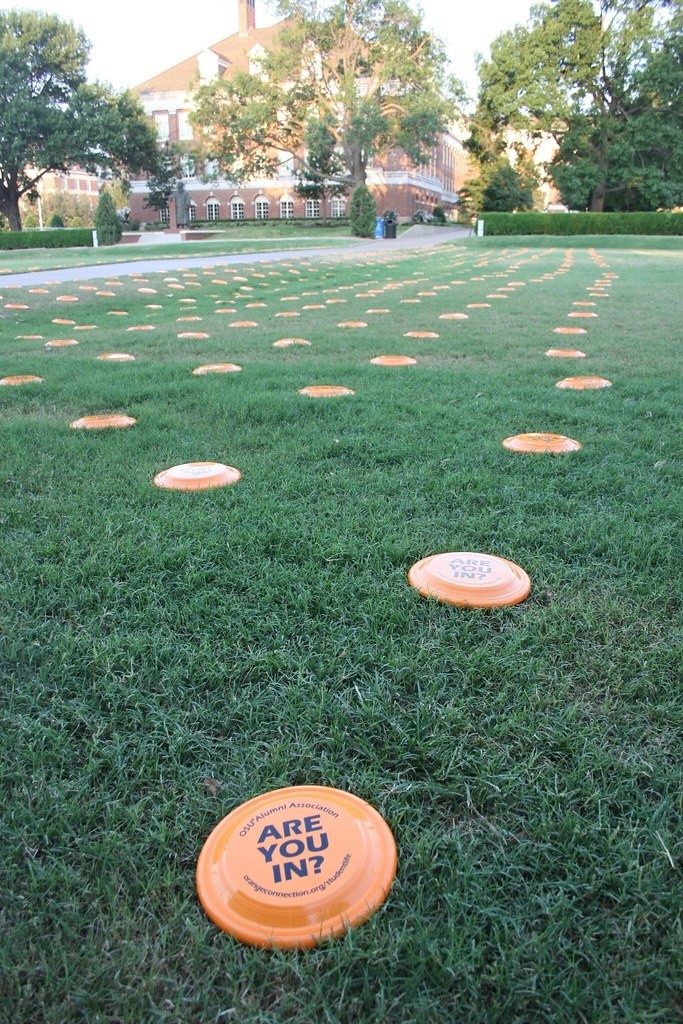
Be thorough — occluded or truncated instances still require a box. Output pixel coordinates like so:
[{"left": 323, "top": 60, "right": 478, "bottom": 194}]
[
  {"left": 173, "top": 182, "right": 192, "bottom": 228},
  {"left": 468, "top": 214, "right": 477, "bottom": 237}
]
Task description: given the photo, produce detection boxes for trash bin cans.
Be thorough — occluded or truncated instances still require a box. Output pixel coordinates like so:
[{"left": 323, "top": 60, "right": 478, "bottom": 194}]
[
  {"left": 374, "top": 218, "right": 385, "bottom": 239},
  {"left": 384, "top": 219, "right": 398, "bottom": 239}
]
